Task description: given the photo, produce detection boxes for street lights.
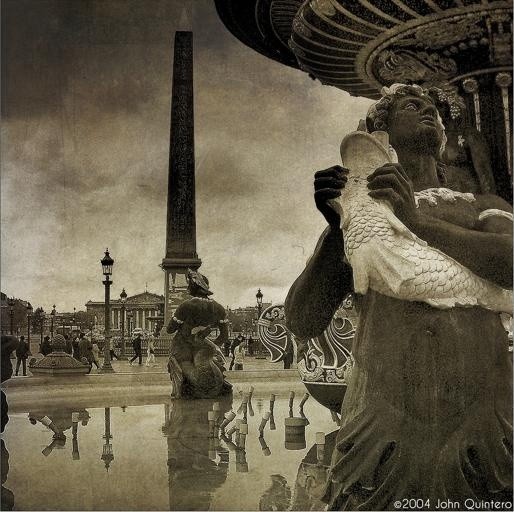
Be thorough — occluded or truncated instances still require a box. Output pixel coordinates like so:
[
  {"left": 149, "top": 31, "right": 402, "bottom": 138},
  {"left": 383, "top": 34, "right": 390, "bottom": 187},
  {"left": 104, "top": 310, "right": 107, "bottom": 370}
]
[
  {"left": 100, "top": 408, "right": 115, "bottom": 472},
  {"left": 256, "top": 288, "right": 264, "bottom": 352},
  {"left": 229, "top": 320, "right": 232, "bottom": 337},
  {"left": 89, "top": 317, "right": 93, "bottom": 344},
  {"left": 52, "top": 304, "right": 56, "bottom": 337},
  {"left": 39, "top": 307, "right": 44, "bottom": 344},
  {"left": 244, "top": 319, "right": 248, "bottom": 350},
  {"left": 26, "top": 303, "right": 33, "bottom": 352},
  {"left": 100, "top": 248, "right": 113, "bottom": 364},
  {"left": 120, "top": 288, "right": 128, "bottom": 354},
  {"left": 8, "top": 297, "right": 16, "bottom": 335}
]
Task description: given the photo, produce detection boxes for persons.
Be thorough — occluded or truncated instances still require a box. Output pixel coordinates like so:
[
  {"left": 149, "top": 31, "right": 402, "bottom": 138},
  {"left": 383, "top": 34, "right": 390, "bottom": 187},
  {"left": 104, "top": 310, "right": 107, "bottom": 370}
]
[
  {"left": 14, "top": 335, "right": 28, "bottom": 376},
  {"left": 145, "top": 337, "right": 156, "bottom": 367},
  {"left": 279, "top": 81, "right": 512, "bottom": 510},
  {"left": 91, "top": 340, "right": 103, "bottom": 369},
  {"left": 228, "top": 333, "right": 243, "bottom": 370},
  {"left": 247, "top": 336, "right": 254, "bottom": 357},
  {"left": 40, "top": 336, "right": 51, "bottom": 354},
  {"left": 72, "top": 337, "right": 80, "bottom": 360},
  {"left": 63, "top": 334, "right": 72, "bottom": 355},
  {"left": 165, "top": 270, "right": 233, "bottom": 398},
  {"left": 109, "top": 335, "right": 119, "bottom": 361},
  {"left": 78, "top": 333, "right": 99, "bottom": 374},
  {"left": 233, "top": 340, "right": 245, "bottom": 370},
  {"left": 128, "top": 334, "right": 144, "bottom": 366},
  {"left": 224, "top": 339, "right": 231, "bottom": 357}
]
